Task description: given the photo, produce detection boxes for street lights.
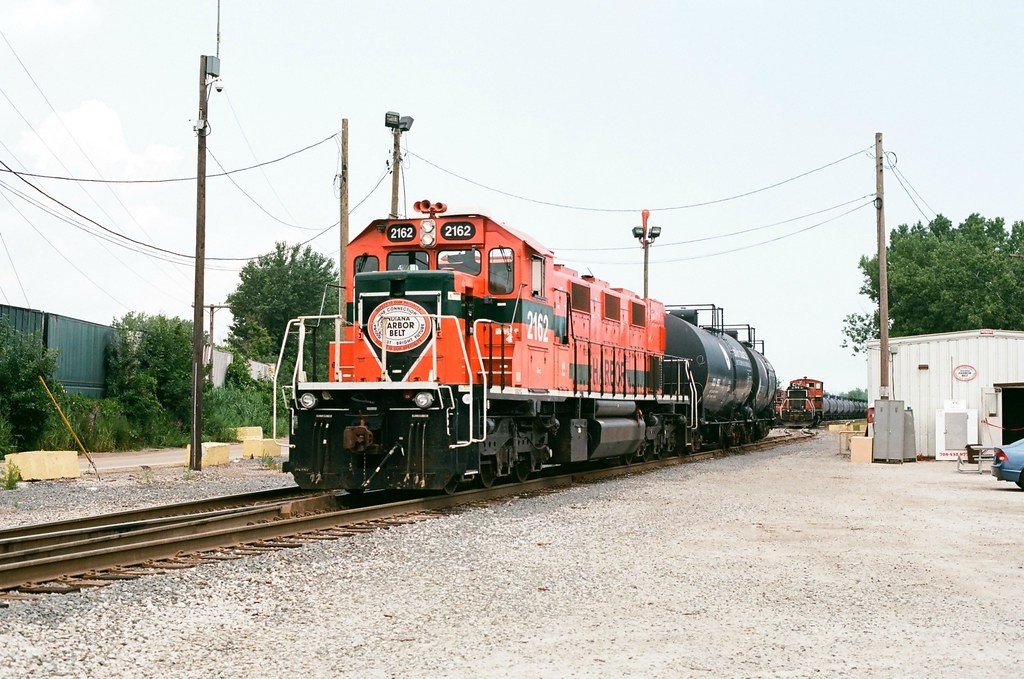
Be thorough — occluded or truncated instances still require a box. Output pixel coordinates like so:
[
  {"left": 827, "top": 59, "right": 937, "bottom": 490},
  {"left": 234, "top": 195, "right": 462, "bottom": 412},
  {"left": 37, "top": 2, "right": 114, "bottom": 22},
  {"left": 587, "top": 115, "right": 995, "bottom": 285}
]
[
  {"left": 382, "top": 110, "right": 414, "bottom": 218},
  {"left": 632, "top": 210, "right": 662, "bottom": 299}
]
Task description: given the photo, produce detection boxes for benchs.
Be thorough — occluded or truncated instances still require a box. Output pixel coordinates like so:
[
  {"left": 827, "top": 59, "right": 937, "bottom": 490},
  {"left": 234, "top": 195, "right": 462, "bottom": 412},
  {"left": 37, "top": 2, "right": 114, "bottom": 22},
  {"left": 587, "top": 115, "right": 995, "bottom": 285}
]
[
  {"left": 973, "top": 458, "right": 994, "bottom": 461},
  {"left": 972, "top": 454, "right": 994, "bottom": 457}
]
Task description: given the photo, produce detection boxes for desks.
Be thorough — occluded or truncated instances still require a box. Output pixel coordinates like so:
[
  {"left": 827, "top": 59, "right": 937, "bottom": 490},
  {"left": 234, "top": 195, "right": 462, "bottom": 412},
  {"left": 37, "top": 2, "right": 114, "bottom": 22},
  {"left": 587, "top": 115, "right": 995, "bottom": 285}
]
[{"left": 961, "top": 446, "right": 995, "bottom": 474}]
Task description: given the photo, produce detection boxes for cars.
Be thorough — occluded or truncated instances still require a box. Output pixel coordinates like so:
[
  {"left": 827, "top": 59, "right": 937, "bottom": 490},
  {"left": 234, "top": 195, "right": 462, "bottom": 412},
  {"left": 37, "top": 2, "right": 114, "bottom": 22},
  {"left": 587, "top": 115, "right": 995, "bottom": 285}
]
[{"left": 992, "top": 437, "right": 1024, "bottom": 489}]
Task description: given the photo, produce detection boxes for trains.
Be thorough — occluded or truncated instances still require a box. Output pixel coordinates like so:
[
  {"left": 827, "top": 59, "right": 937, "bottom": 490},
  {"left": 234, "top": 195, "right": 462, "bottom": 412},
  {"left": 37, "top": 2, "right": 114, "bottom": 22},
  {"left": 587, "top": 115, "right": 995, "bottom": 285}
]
[
  {"left": 272, "top": 200, "right": 780, "bottom": 493},
  {"left": 775, "top": 376, "right": 868, "bottom": 426}
]
